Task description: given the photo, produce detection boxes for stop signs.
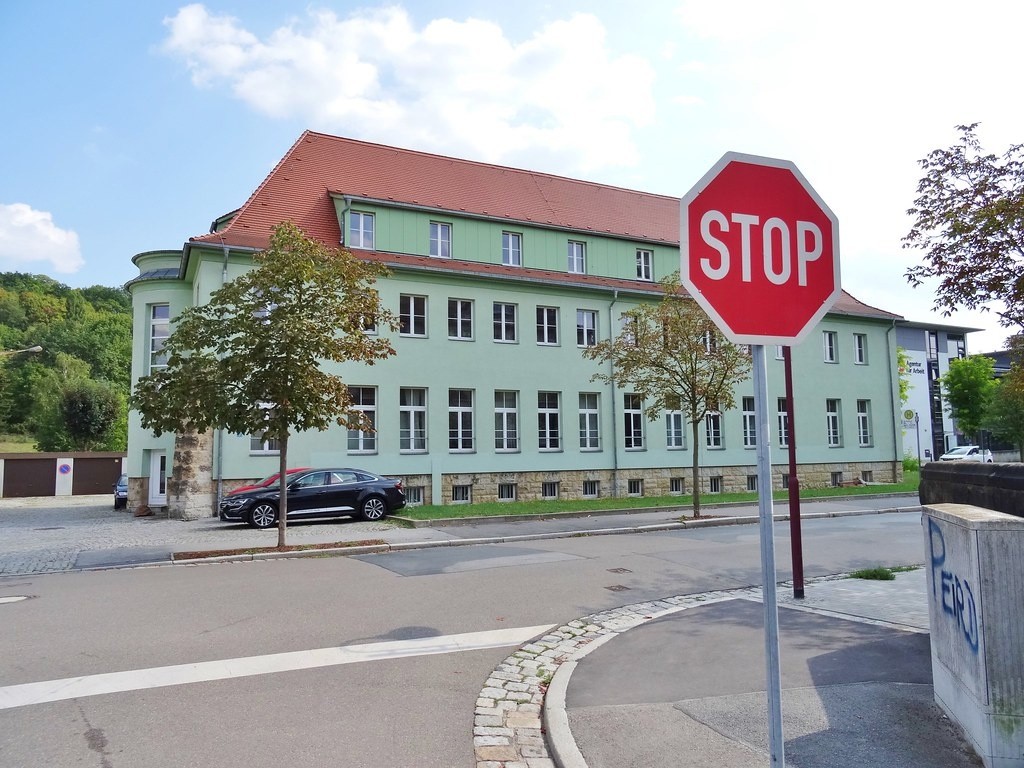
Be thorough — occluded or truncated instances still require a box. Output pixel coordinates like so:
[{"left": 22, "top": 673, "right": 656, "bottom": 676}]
[{"left": 682, "top": 149, "right": 842, "bottom": 350}]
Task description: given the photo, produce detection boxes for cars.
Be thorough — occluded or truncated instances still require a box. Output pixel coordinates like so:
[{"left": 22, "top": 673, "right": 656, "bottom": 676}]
[
  {"left": 112, "top": 474, "right": 127, "bottom": 507},
  {"left": 220, "top": 468, "right": 406, "bottom": 533}
]
